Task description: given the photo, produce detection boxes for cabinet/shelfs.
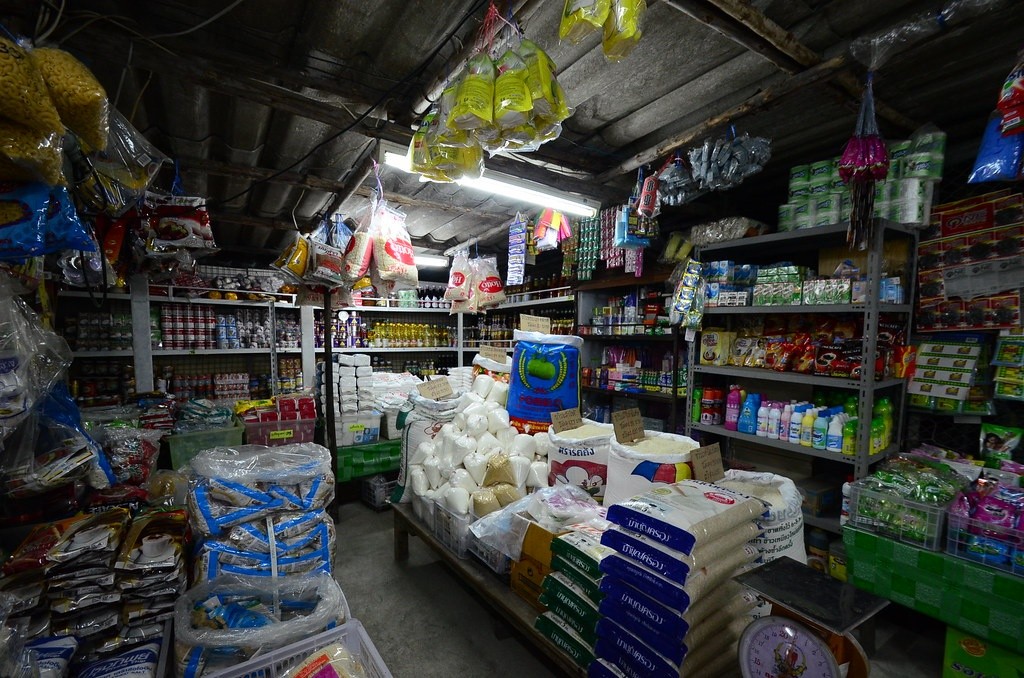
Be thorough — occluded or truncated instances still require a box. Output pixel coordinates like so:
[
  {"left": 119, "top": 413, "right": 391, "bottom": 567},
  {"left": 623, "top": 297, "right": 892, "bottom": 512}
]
[
  {"left": 48, "top": 283, "right": 576, "bottom": 403},
  {"left": 575, "top": 270, "right": 690, "bottom": 434},
  {"left": 686, "top": 218, "right": 920, "bottom": 538}
]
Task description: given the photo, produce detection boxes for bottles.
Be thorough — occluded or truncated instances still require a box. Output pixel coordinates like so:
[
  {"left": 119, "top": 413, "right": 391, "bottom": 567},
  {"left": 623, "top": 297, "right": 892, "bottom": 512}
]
[
  {"left": 362, "top": 321, "right": 458, "bottom": 347},
  {"left": 410, "top": 283, "right": 452, "bottom": 309},
  {"left": 371, "top": 353, "right": 458, "bottom": 381},
  {"left": 463, "top": 309, "right": 577, "bottom": 347},
  {"left": 500, "top": 270, "right": 576, "bottom": 303}
]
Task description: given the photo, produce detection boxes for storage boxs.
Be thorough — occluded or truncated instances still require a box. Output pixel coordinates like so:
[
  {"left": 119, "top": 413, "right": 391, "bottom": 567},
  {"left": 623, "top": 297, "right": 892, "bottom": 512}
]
[
  {"left": 697, "top": 257, "right": 759, "bottom": 307},
  {"left": 852, "top": 273, "right": 906, "bottom": 305},
  {"left": 724, "top": 433, "right": 841, "bottom": 519},
  {"left": 199, "top": 577, "right": 394, "bottom": 678},
  {"left": 164, "top": 404, "right": 319, "bottom": 471},
  {"left": 701, "top": 330, "right": 738, "bottom": 368},
  {"left": 843, "top": 478, "right": 1024, "bottom": 678},
  {"left": 432, "top": 497, "right": 576, "bottom": 615},
  {"left": 361, "top": 471, "right": 399, "bottom": 510}
]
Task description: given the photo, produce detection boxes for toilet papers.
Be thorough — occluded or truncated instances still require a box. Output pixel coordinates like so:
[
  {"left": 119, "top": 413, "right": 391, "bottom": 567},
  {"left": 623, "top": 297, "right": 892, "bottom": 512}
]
[{"left": 779, "top": 131, "right": 946, "bottom": 229}]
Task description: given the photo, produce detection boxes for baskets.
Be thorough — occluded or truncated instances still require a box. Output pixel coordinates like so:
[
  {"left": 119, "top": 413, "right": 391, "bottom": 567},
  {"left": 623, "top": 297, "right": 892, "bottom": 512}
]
[{"left": 203, "top": 619, "right": 393, "bottom": 678}]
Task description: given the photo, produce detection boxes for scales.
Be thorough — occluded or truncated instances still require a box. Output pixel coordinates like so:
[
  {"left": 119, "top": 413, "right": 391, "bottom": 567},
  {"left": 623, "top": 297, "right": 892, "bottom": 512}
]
[{"left": 729, "top": 556, "right": 891, "bottom": 678}]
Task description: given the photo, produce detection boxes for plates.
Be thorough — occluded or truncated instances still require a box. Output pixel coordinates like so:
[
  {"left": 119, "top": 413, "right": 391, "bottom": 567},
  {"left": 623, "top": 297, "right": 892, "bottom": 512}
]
[
  {"left": 127, "top": 546, "right": 176, "bottom": 565},
  {"left": 57, "top": 531, "right": 110, "bottom": 552}
]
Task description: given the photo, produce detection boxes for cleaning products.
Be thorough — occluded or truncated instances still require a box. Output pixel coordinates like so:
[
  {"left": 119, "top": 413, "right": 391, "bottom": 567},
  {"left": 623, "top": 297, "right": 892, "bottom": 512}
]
[{"left": 693, "top": 383, "right": 893, "bottom": 456}]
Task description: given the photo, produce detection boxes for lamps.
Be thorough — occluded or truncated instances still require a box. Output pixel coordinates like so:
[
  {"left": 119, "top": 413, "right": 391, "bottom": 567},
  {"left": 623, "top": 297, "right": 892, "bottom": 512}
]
[
  {"left": 411, "top": 252, "right": 449, "bottom": 268},
  {"left": 382, "top": 148, "right": 602, "bottom": 221}
]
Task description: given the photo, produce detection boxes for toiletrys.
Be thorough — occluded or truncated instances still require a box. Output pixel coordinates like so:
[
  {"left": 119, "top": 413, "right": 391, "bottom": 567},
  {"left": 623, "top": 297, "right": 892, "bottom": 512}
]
[{"left": 704, "top": 259, "right": 903, "bottom": 307}]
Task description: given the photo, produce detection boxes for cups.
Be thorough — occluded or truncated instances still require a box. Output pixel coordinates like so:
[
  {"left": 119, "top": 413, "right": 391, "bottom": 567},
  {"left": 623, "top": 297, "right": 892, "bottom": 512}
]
[
  {"left": 141, "top": 533, "right": 174, "bottom": 558},
  {"left": 73, "top": 526, "right": 102, "bottom": 544}
]
